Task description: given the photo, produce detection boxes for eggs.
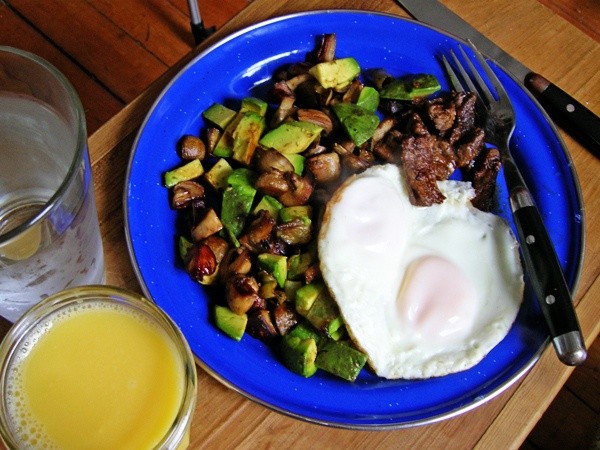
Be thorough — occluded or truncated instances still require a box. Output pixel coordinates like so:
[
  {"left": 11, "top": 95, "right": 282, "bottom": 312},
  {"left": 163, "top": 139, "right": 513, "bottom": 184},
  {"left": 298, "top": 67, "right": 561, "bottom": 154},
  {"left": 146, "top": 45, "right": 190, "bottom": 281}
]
[{"left": 318, "top": 163, "right": 524, "bottom": 377}]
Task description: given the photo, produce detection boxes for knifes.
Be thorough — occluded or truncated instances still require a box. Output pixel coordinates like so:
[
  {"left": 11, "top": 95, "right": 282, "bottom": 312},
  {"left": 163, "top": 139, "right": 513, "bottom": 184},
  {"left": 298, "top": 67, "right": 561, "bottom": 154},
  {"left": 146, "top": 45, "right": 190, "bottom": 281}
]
[{"left": 394, "top": 0, "right": 599, "bottom": 161}]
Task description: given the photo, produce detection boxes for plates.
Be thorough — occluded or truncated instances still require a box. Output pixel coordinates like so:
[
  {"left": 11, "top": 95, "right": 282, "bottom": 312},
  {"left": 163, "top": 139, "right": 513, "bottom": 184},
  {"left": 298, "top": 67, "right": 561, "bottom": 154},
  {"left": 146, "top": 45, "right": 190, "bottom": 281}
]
[{"left": 123, "top": 10, "right": 583, "bottom": 429}]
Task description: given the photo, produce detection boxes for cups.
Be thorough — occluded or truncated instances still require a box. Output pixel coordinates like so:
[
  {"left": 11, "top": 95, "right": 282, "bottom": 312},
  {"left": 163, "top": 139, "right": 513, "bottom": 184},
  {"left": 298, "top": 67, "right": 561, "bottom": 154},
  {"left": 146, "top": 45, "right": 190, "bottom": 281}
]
[
  {"left": 0, "top": 284, "right": 199, "bottom": 450},
  {"left": 0, "top": 44, "right": 106, "bottom": 324}
]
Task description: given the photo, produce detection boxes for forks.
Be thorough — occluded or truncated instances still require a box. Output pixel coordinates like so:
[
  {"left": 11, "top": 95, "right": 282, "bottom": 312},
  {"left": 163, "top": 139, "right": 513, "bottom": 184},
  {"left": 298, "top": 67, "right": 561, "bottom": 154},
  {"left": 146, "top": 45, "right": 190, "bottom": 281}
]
[{"left": 437, "top": 37, "right": 590, "bottom": 368}]
